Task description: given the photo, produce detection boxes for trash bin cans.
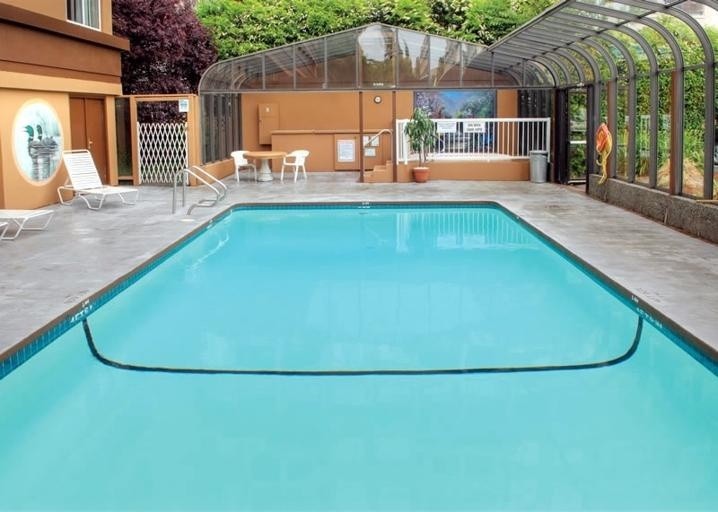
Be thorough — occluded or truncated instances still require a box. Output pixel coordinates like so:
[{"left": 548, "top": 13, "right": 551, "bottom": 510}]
[{"left": 529, "top": 150, "right": 548, "bottom": 183}]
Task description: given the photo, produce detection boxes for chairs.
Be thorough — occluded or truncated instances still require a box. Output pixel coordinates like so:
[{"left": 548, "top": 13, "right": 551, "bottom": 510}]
[
  {"left": 231, "top": 149, "right": 257, "bottom": 183},
  {"left": 0, "top": 209, "right": 55, "bottom": 239},
  {"left": 280, "top": 149, "right": 310, "bottom": 183},
  {"left": 57, "top": 149, "right": 140, "bottom": 209}
]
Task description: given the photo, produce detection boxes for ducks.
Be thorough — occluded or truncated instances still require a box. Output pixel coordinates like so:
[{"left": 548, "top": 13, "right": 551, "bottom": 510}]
[{"left": 21, "top": 125, "right": 59, "bottom": 148}]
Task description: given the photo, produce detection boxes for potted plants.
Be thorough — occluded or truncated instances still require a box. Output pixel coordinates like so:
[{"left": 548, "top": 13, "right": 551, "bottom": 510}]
[{"left": 402, "top": 108, "right": 438, "bottom": 183}]
